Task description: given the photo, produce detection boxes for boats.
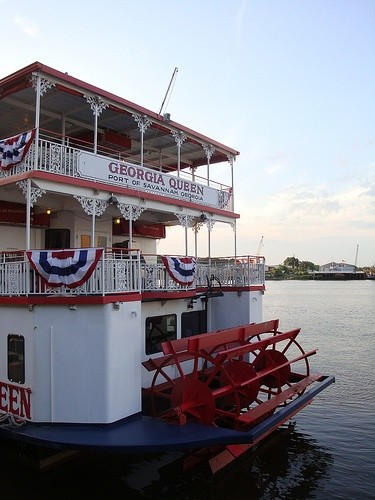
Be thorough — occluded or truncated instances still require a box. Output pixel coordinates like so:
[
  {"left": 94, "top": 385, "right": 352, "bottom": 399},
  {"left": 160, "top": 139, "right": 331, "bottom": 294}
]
[{"left": 1, "top": 63, "right": 334, "bottom": 481}]
